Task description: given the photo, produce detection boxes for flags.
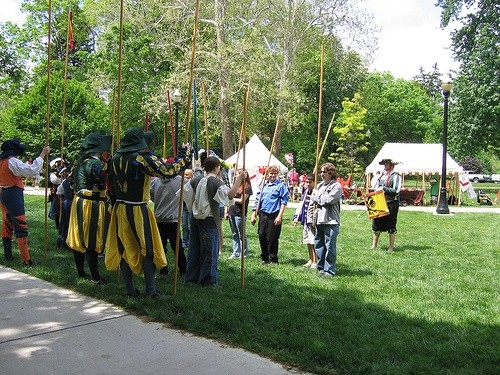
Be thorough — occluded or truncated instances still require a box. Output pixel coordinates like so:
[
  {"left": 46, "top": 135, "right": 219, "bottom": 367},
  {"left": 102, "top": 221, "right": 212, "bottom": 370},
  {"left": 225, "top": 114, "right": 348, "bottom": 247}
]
[{"left": 69, "top": 11, "right": 76, "bottom": 49}]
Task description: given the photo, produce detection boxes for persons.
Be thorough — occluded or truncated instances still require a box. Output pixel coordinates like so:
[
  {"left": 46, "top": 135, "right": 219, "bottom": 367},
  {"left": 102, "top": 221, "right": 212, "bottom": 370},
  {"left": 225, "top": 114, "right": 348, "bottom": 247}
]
[
  {"left": 226, "top": 168, "right": 253, "bottom": 259},
  {"left": 292, "top": 173, "right": 318, "bottom": 270},
  {"left": 287, "top": 167, "right": 308, "bottom": 194},
  {"left": 371, "top": 159, "right": 401, "bottom": 253},
  {"left": 428, "top": 175, "right": 441, "bottom": 206},
  {"left": 24, "top": 155, "right": 39, "bottom": 190},
  {"left": 0, "top": 140, "right": 51, "bottom": 266},
  {"left": 173, "top": 148, "right": 248, "bottom": 287},
  {"left": 49, "top": 127, "right": 194, "bottom": 298},
  {"left": 311, "top": 163, "right": 342, "bottom": 277},
  {"left": 251, "top": 165, "right": 289, "bottom": 265}
]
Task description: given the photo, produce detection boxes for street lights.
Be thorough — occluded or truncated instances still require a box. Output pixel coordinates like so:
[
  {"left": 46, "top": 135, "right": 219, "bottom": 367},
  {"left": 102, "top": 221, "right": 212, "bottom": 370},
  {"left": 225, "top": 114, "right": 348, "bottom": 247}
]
[
  {"left": 435, "top": 81, "right": 454, "bottom": 214},
  {"left": 365, "top": 129, "right": 371, "bottom": 166},
  {"left": 172, "top": 89, "right": 182, "bottom": 158}
]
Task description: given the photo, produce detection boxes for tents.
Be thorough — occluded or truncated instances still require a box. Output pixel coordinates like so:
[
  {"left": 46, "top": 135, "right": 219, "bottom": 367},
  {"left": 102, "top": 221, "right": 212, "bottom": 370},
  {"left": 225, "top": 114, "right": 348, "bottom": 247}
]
[
  {"left": 365, "top": 143, "right": 464, "bottom": 207},
  {"left": 224, "top": 133, "right": 289, "bottom": 200}
]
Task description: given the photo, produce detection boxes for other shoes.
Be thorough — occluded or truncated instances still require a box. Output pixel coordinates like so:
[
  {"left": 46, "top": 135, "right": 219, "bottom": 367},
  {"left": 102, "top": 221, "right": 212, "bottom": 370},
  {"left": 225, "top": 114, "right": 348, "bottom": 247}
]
[
  {"left": 7, "top": 257, "right": 17, "bottom": 260},
  {"left": 23, "top": 260, "right": 36, "bottom": 266},
  {"left": 303, "top": 260, "right": 317, "bottom": 269},
  {"left": 228, "top": 253, "right": 235, "bottom": 259},
  {"left": 91, "top": 279, "right": 107, "bottom": 285},
  {"left": 80, "top": 275, "right": 91, "bottom": 281},
  {"left": 130, "top": 288, "right": 140, "bottom": 297},
  {"left": 153, "top": 290, "right": 161, "bottom": 297}
]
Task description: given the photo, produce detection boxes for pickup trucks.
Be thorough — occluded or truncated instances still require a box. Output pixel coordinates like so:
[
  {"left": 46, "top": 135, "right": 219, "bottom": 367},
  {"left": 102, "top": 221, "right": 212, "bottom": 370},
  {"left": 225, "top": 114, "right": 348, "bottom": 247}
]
[{"left": 463, "top": 171, "right": 490, "bottom": 183}]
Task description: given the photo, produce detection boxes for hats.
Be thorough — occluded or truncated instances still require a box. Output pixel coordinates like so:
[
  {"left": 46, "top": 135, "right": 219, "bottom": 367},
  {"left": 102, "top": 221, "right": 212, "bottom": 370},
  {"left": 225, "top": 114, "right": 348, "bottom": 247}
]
[
  {"left": 79, "top": 131, "right": 113, "bottom": 155},
  {"left": 0, "top": 140, "right": 25, "bottom": 158},
  {"left": 116, "top": 127, "right": 155, "bottom": 154},
  {"left": 379, "top": 158, "right": 399, "bottom": 165}
]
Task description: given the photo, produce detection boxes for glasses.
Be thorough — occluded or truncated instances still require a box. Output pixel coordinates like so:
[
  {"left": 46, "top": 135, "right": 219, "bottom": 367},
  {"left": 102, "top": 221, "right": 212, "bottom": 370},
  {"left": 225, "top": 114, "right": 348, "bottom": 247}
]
[{"left": 320, "top": 171, "right": 327, "bottom": 174}]
